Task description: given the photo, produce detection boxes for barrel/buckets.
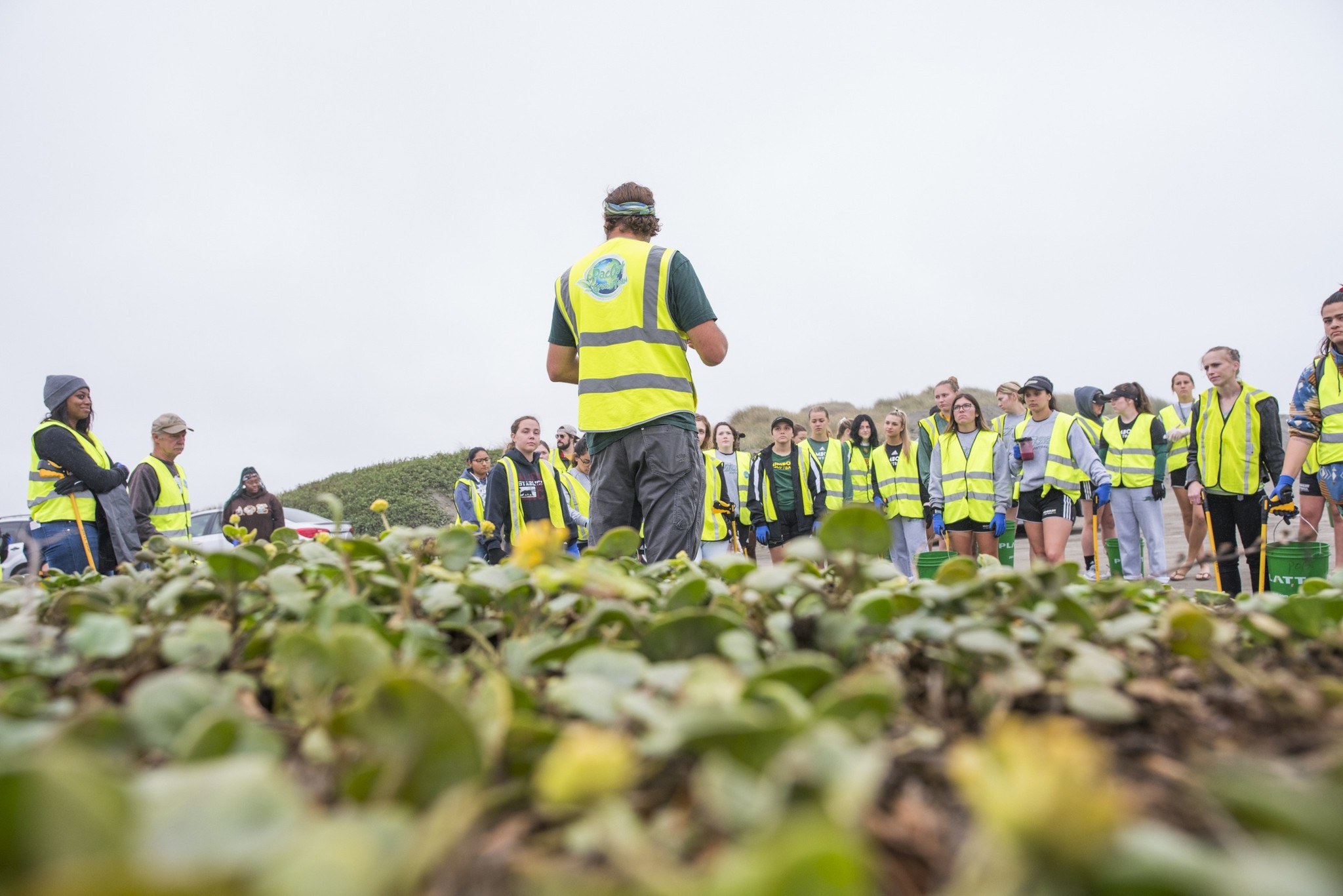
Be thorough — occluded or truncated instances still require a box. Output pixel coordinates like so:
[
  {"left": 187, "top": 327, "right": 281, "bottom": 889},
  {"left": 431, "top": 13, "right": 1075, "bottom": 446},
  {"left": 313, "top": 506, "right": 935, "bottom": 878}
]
[
  {"left": 1106, "top": 538, "right": 1144, "bottom": 577},
  {"left": 1326, "top": 499, "right": 1334, "bottom": 527},
  {"left": 976, "top": 520, "right": 1015, "bottom": 567},
  {"left": 913, "top": 529, "right": 958, "bottom": 579},
  {"left": 1266, "top": 514, "right": 1331, "bottom": 596}
]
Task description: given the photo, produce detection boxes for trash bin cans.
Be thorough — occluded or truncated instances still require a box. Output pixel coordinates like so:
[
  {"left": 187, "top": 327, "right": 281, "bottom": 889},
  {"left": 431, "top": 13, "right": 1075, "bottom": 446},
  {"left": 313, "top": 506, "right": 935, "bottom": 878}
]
[
  {"left": 1266, "top": 540, "right": 1330, "bottom": 596},
  {"left": 1106, "top": 537, "right": 1144, "bottom": 579},
  {"left": 998, "top": 520, "right": 1017, "bottom": 565},
  {"left": 914, "top": 551, "right": 958, "bottom": 580}
]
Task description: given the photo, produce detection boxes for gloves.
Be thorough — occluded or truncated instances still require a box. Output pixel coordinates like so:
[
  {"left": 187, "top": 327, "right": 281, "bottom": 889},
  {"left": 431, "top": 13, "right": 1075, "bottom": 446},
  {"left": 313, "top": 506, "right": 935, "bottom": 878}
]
[
  {"left": 1013, "top": 444, "right": 1022, "bottom": 460},
  {"left": 932, "top": 513, "right": 945, "bottom": 535},
  {"left": 923, "top": 506, "right": 932, "bottom": 528},
  {"left": 1, "top": 533, "right": 12, "bottom": 551},
  {"left": 874, "top": 496, "right": 888, "bottom": 515},
  {"left": 54, "top": 475, "right": 89, "bottom": 495},
  {"left": 755, "top": 526, "right": 770, "bottom": 546},
  {"left": 989, "top": 513, "right": 1006, "bottom": 538},
  {"left": 568, "top": 541, "right": 580, "bottom": 559},
  {"left": 1090, "top": 484, "right": 1112, "bottom": 508},
  {"left": 114, "top": 462, "right": 130, "bottom": 480},
  {"left": 1152, "top": 480, "right": 1166, "bottom": 501},
  {"left": 812, "top": 521, "right": 823, "bottom": 538},
  {"left": 1269, "top": 474, "right": 1295, "bottom": 516},
  {"left": 488, "top": 549, "right": 502, "bottom": 566}
]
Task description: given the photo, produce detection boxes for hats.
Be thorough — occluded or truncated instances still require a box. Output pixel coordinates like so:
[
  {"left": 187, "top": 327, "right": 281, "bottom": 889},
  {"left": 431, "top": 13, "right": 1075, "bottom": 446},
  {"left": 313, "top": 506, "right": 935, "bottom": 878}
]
[
  {"left": 44, "top": 374, "right": 90, "bottom": 412},
  {"left": 735, "top": 429, "right": 746, "bottom": 438},
  {"left": 1017, "top": 378, "right": 1053, "bottom": 395},
  {"left": 1101, "top": 388, "right": 1138, "bottom": 402},
  {"left": 152, "top": 413, "right": 194, "bottom": 434},
  {"left": 1093, "top": 393, "right": 1111, "bottom": 404},
  {"left": 771, "top": 416, "right": 794, "bottom": 428},
  {"left": 557, "top": 425, "right": 581, "bottom": 440}
]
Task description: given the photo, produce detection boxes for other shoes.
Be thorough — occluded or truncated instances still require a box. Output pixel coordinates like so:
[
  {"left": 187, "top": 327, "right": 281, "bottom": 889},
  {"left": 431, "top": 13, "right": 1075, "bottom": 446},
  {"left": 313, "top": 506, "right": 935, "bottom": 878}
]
[{"left": 1083, "top": 565, "right": 1111, "bottom": 581}]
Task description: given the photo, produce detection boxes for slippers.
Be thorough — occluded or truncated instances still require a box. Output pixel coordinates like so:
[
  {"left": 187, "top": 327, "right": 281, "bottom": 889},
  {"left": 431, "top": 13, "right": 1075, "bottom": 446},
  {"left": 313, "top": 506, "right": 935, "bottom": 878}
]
[
  {"left": 1170, "top": 567, "right": 1185, "bottom": 581},
  {"left": 1196, "top": 572, "right": 1211, "bottom": 580}
]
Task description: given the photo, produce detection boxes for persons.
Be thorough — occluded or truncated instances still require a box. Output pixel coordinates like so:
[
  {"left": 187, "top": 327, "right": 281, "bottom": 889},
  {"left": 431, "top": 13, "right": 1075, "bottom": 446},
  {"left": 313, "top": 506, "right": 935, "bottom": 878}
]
[
  {"left": 745, "top": 418, "right": 828, "bottom": 571},
  {"left": 127, "top": 413, "right": 198, "bottom": 569},
  {"left": 1157, "top": 371, "right": 1213, "bottom": 583},
  {"left": 708, "top": 422, "right": 758, "bottom": 572},
  {"left": 692, "top": 413, "right": 732, "bottom": 569},
  {"left": 453, "top": 446, "right": 492, "bottom": 561},
  {"left": 1186, "top": 345, "right": 1301, "bottom": 601},
  {"left": 928, "top": 395, "right": 1013, "bottom": 565},
  {"left": 1267, "top": 287, "right": 1343, "bottom": 519},
  {"left": 1098, "top": 380, "right": 1168, "bottom": 582},
  {"left": 1006, "top": 375, "right": 1112, "bottom": 584},
  {"left": 871, "top": 408, "right": 929, "bottom": 577},
  {"left": 990, "top": 381, "right": 1040, "bottom": 577},
  {"left": 547, "top": 183, "right": 729, "bottom": 560},
  {"left": 1296, "top": 432, "right": 1343, "bottom": 571},
  {"left": 920, "top": 377, "right": 964, "bottom": 555},
  {"left": 534, "top": 423, "right": 590, "bottom": 551},
  {"left": 792, "top": 404, "right": 880, "bottom": 543},
  {"left": 222, "top": 468, "right": 286, "bottom": 546},
  {"left": 1074, "top": 385, "right": 1118, "bottom": 577},
  {"left": 483, "top": 415, "right": 580, "bottom": 568},
  {"left": 26, "top": 374, "right": 130, "bottom": 585}
]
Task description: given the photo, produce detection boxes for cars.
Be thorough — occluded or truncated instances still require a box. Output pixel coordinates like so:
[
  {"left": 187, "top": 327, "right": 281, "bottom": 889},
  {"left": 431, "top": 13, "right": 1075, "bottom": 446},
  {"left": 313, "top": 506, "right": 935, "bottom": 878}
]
[
  {"left": 191, "top": 507, "right": 353, "bottom": 553},
  {"left": 0, "top": 514, "right": 32, "bottom": 581}
]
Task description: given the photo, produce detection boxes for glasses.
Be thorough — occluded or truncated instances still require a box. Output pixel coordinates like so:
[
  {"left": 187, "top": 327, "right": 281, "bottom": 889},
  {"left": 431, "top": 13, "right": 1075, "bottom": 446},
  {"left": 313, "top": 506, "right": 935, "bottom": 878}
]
[
  {"left": 952, "top": 403, "right": 972, "bottom": 411},
  {"left": 555, "top": 434, "right": 564, "bottom": 439},
  {"left": 577, "top": 455, "right": 590, "bottom": 464},
  {"left": 471, "top": 458, "right": 492, "bottom": 462}
]
